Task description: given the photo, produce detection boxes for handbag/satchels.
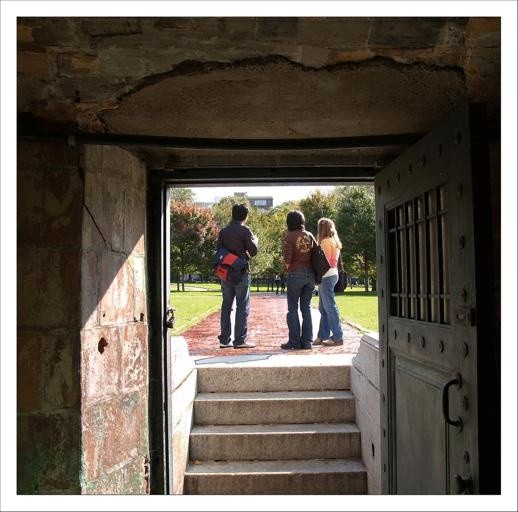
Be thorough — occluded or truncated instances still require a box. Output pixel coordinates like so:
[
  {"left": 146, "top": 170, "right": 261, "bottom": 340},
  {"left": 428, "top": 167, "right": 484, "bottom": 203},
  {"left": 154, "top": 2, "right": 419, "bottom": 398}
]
[
  {"left": 335, "top": 271, "right": 347, "bottom": 293},
  {"left": 214, "top": 248, "right": 247, "bottom": 287},
  {"left": 310, "top": 246, "right": 331, "bottom": 283}
]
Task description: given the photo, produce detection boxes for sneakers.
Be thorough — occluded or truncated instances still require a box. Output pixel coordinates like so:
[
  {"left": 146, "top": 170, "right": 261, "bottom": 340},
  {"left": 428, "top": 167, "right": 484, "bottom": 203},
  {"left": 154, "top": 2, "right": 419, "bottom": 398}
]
[
  {"left": 220, "top": 339, "right": 257, "bottom": 349},
  {"left": 281, "top": 337, "right": 344, "bottom": 349}
]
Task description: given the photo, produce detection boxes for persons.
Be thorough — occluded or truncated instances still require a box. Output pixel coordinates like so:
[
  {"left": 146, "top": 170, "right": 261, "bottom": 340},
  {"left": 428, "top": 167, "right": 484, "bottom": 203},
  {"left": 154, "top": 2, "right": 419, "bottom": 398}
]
[
  {"left": 281, "top": 273, "right": 286, "bottom": 294},
  {"left": 216, "top": 203, "right": 257, "bottom": 348},
  {"left": 279, "top": 210, "right": 317, "bottom": 349},
  {"left": 275, "top": 271, "right": 281, "bottom": 295},
  {"left": 311, "top": 217, "right": 343, "bottom": 345}
]
